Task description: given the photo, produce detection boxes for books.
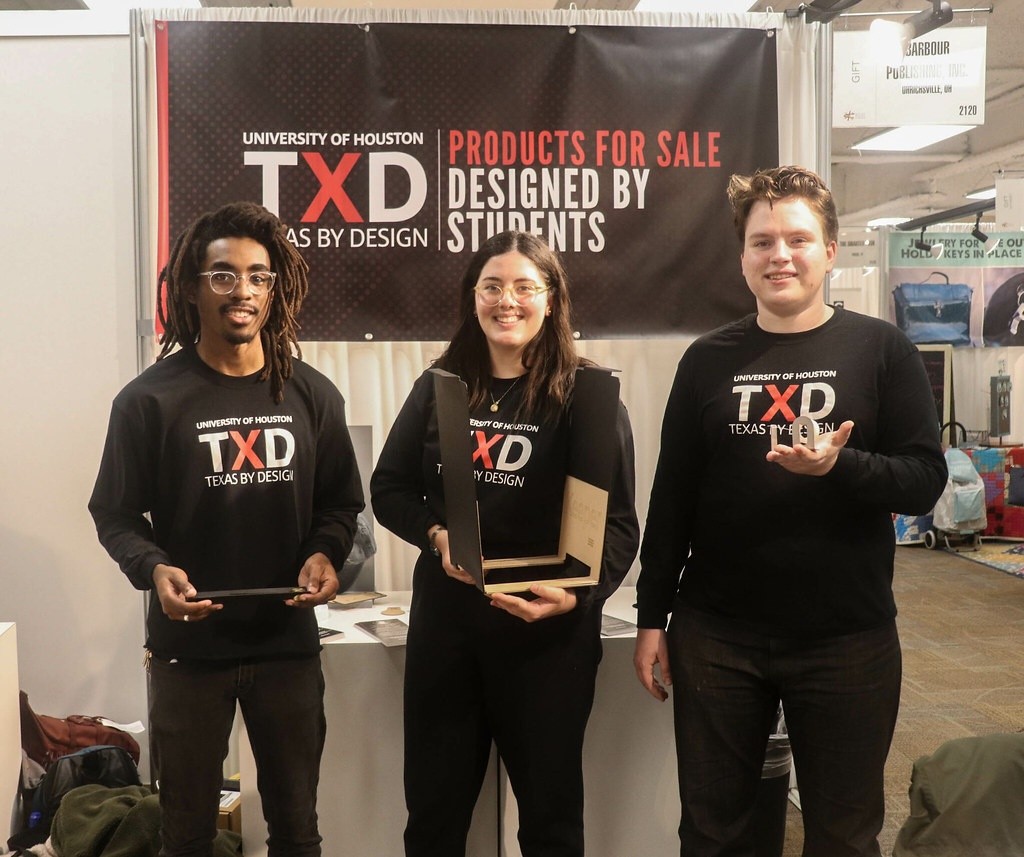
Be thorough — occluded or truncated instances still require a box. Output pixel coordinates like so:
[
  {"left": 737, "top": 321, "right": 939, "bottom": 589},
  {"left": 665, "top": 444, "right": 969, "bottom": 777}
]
[
  {"left": 317, "top": 626, "right": 344, "bottom": 644},
  {"left": 601, "top": 614, "right": 636, "bottom": 635},
  {"left": 355, "top": 619, "right": 409, "bottom": 647}
]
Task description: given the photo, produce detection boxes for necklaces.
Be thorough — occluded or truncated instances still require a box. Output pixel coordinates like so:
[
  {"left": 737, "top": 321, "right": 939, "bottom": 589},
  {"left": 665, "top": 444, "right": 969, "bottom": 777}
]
[{"left": 484, "top": 374, "right": 522, "bottom": 411}]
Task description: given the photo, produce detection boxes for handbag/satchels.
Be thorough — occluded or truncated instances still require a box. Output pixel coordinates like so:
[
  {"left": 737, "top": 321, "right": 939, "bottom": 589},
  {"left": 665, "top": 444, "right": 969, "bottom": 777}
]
[{"left": 893, "top": 271, "right": 975, "bottom": 345}]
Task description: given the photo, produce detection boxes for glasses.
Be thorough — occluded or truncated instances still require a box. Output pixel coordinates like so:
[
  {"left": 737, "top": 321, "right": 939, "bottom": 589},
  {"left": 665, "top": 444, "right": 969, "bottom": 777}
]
[
  {"left": 472, "top": 282, "right": 553, "bottom": 307},
  {"left": 196, "top": 271, "right": 277, "bottom": 295}
]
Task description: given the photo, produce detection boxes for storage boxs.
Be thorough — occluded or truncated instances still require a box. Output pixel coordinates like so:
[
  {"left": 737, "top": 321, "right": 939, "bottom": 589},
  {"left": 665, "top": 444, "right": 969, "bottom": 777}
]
[{"left": 218, "top": 789, "right": 241, "bottom": 833}]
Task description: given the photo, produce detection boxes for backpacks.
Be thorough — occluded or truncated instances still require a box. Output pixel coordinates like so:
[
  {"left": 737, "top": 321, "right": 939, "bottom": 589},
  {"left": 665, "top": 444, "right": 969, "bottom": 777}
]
[{"left": 7, "top": 689, "right": 143, "bottom": 857}]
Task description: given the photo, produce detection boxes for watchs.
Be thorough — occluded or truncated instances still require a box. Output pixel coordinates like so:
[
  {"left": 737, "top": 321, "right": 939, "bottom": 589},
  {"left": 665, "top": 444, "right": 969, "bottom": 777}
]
[{"left": 429, "top": 527, "right": 445, "bottom": 556}]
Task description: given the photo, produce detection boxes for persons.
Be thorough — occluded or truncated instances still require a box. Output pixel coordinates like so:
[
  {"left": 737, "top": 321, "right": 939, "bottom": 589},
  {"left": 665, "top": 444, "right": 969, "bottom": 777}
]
[
  {"left": 371, "top": 231, "right": 640, "bottom": 857},
  {"left": 632, "top": 165, "right": 948, "bottom": 857},
  {"left": 87, "top": 203, "right": 366, "bottom": 857}
]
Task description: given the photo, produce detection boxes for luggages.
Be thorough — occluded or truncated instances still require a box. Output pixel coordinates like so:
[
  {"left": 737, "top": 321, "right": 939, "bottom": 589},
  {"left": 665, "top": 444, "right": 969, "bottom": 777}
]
[{"left": 925, "top": 422, "right": 987, "bottom": 552}]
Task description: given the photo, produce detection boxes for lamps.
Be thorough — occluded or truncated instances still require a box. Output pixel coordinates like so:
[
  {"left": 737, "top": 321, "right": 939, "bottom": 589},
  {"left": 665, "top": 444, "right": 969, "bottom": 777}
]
[
  {"left": 870, "top": 0, "right": 954, "bottom": 69},
  {"left": 971, "top": 211, "right": 1000, "bottom": 254},
  {"left": 915, "top": 226, "right": 944, "bottom": 259}
]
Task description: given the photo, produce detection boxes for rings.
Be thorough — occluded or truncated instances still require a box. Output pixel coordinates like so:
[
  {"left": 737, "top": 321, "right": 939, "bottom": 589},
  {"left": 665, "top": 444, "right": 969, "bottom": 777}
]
[{"left": 184, "top": 615, "right": 189, "bottom": 622}]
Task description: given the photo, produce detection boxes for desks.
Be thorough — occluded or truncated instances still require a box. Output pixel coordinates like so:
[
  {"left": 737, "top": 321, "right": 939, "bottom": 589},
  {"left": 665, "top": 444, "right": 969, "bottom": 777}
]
[{"left": 240, "top": 590, "right": 681, "bottom": 856}]
